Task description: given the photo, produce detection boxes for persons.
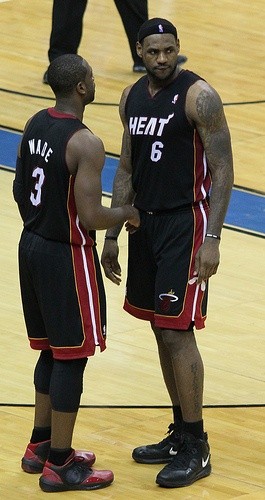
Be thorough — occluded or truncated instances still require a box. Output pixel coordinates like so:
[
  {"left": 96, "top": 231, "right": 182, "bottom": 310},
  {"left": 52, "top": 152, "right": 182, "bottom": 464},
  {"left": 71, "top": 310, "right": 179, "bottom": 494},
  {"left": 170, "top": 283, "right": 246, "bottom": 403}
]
[
  {"left": 12, "top": 54, "right": 114, "bottom": 492},
  {"left": 101, "top": 17, "right": 234, "bottom": 490},
  {"left": 43, "top": 0, "right": 188, "bottom": 83}
]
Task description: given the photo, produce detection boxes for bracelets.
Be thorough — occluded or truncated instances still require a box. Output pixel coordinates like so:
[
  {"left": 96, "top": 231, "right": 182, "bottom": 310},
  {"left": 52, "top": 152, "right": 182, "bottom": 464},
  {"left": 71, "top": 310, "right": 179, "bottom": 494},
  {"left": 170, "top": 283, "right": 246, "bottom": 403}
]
[
  {"left": 205, "top": 233, "right": 221, "bottom": 241},
  {"left": 104, "top": 236, "right": 118, "bottom": 241}
]
